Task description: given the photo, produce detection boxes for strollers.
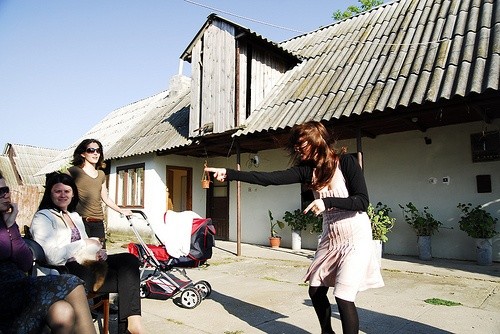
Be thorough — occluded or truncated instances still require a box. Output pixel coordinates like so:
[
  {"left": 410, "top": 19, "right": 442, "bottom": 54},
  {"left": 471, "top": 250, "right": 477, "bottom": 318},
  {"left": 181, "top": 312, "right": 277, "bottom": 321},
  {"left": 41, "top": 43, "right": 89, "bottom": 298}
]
[{"left": 121, "top": 209, "right": 216, "bottom": 309}]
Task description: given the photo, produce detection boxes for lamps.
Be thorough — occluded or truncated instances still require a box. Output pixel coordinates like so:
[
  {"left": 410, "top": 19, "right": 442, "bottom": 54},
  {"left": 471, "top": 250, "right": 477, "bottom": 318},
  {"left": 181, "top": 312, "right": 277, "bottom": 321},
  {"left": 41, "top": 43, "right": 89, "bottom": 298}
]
[
  {"left": 457, "top": 203, "right": 499, "bottom": 265},
  {"left": 368, "top": 202, "right": 396, "bottom": 258}
]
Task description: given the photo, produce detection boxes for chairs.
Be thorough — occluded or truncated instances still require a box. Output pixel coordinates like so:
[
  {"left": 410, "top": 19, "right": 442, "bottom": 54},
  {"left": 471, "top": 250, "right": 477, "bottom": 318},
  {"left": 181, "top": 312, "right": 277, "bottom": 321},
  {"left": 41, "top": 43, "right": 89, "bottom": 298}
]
[{"left": 24, "top": 224, "right": 109, "bottom": 334}]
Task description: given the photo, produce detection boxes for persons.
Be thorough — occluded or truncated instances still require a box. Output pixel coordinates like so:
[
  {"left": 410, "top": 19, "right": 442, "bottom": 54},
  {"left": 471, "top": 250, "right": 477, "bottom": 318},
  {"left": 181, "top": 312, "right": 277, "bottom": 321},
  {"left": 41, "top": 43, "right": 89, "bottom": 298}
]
[
  {"left": 31, "top": 172, "right": 141, "bottom": 334},
  {"left": 205, "top": 121, "right": 385, "bottom": 334},
  {"left": 0, "top": 172, "right": 97, "bottom": 334},
  {"left": 66, "top": 139, "right": 133, "bottom": 250}
]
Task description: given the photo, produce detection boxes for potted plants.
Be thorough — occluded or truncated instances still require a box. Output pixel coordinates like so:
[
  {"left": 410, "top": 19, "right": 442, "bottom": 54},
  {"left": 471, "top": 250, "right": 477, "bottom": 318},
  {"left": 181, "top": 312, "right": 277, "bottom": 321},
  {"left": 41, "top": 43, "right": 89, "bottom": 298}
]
[
  {"left": 268, "top": 209, "right": 285, "bottom": 247},
  {"left": 283, "top": 209, "right": 307, "bottom": 249},
  {"left": 400, "top": 202, "right": 443, "bottom": 261},
  {"left": 309, "top": 212, "right": 323, "bottom": 245},
  {"left": 201, "top": 160, "right": 210, "bottom": 189}
]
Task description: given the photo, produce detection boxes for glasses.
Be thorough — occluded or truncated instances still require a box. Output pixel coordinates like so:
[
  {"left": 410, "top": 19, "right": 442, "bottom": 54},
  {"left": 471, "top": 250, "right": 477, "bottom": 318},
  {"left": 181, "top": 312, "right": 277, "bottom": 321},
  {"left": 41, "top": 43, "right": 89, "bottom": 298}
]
[
  {"left": 87, "top": 148, "right": 102, "bottom": 153},
  {"left": 0, "top": 186, "right": 10, "bottom": 193}
]
